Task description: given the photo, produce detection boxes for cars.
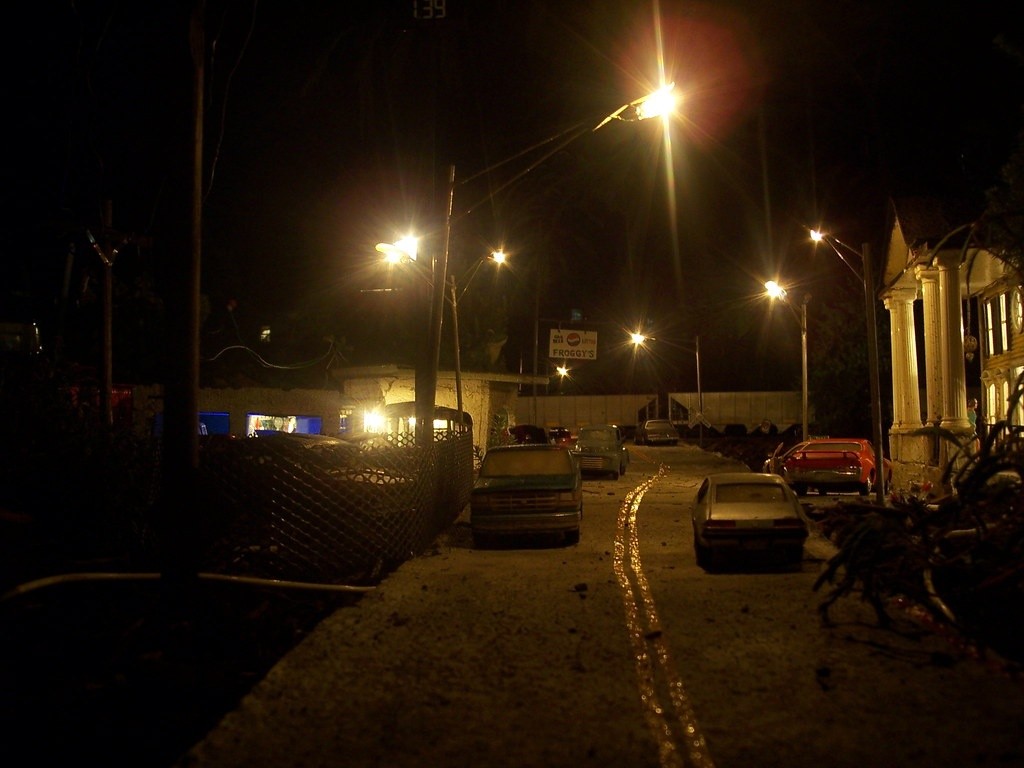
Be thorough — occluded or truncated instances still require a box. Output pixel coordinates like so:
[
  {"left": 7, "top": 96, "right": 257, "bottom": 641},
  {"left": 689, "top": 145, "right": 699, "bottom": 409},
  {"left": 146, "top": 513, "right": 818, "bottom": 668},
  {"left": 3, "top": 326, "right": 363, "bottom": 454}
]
[
  {"left": 764, "top": 438, "right": 875, "bottom": 496},
  {"left": 631, "top": 418, "right": 679, "bottom": 445},
  {"left": 572, "top": 423, "right": 629, "bottom": 479},
  {"left": 471, "top": 446, "right": 585, "bottom": 546},
  {"left": 31, "top": 430, "right": 431, "bottom": 582},
  {"left": 692, "top": 473, "right": 809, "bottom": 571},
  {"left": 501, "top": 423, "right": 547, "bottom": 445}
]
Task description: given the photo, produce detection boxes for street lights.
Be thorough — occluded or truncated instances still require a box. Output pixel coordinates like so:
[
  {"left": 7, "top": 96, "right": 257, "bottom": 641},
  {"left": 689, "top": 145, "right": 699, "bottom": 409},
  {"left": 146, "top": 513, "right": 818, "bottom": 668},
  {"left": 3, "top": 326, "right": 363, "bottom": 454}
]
[
  {"left": 765, "top": 281, "right": 809, "bottom": 438},
  {"left": 630, "top": 331, "right": 703, "bottom": 414},
  {"left": 374, "top": 244, "right": 505, "bottom": 432},
  {"left": 413, "top": 84, "right": 680, "bottom": 445},
  {"left": 810, "top": 228, "right": 885, "bottom": 507}
]
